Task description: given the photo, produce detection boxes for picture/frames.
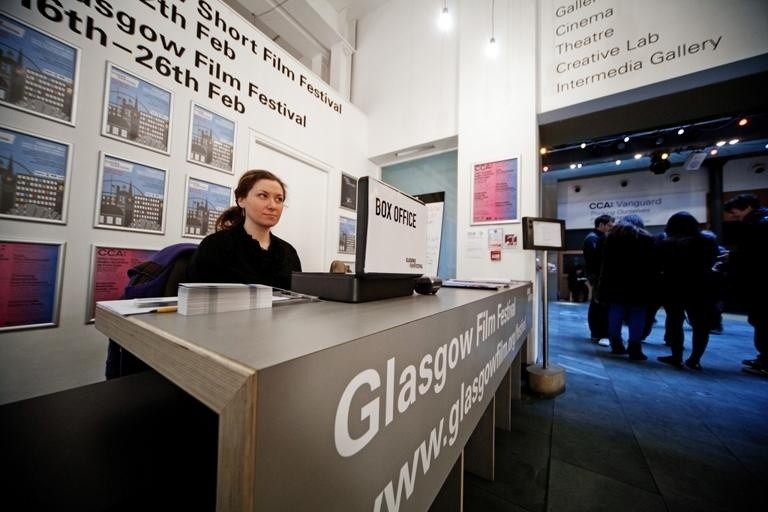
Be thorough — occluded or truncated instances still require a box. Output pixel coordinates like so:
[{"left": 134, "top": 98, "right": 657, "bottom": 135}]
[{"left": 470, "top": 156, "right": 521, "bottom": 226}]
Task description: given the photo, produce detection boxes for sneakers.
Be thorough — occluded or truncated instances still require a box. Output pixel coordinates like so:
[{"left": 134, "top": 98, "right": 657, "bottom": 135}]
[
  {"left": 685, "top": 359, "right": 701, "bottom": 369},
  {"left": 657, "top": 355, "right": 685, "bottom": 368},
  {"left": 742, "top": 359, "right": 768, "bottom": 379},
  {"left": 590, "top": 333, "right": 648, "bottom": 362}
]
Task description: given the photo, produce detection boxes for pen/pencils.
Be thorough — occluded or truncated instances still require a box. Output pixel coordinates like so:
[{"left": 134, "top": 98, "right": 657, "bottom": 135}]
[{"left": 151, "top": 307, "right": 177, "bottom": 314}]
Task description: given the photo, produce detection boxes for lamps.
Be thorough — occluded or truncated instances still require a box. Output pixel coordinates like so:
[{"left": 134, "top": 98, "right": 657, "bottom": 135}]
[
  {"left": 479, "top": 0, "right": 506, "bottom": 65},
  {"left": 438, "top": 0, "right": 451, "bottom": 31},
  {"left": 539, "top": 116, "right": 748, "bottom": 174}
]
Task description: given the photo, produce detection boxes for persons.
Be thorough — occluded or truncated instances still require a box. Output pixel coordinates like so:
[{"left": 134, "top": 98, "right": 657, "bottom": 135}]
[
  {"left": 582, "top": 193, "right": 768, "bottom": 377},
  {"left": 185, "top": 169, "right": 302, "bottom": 295}
]
